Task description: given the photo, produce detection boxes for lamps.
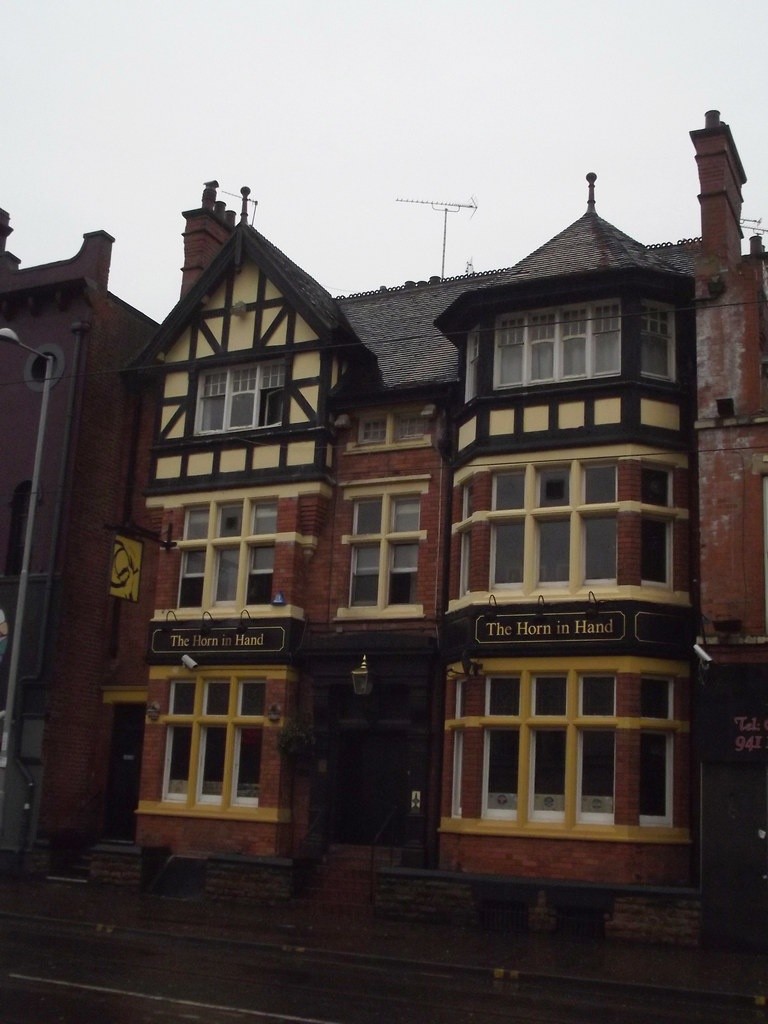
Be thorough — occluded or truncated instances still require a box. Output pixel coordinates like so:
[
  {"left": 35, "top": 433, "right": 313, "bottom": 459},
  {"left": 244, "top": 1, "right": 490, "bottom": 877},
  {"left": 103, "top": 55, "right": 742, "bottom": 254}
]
[
  {"left": 420, "top": 403, "right": 437, "bottom": 419},
  {"left": 716, "top": 398, "right": 735, "bottom": 416},
  {"left": 351, "top": 655, "right": 377, "bottom": 697},
  {"left": 334, "top": 414, "right": 351, "bottom": 428},
  {"left": 268, "top": 701, "right": 282, "bottom": 723},
  {"left": 198, "top": 611, "right": 219, "bottom": 635},
  {"left": 235, "top": 610, "right": 256, "bottom": 635},
  {"left": 483, "top": 595, "right": 502, "bottom": 623},
  {"left": 148, "top": 701, "right": 160, "bottom": 721},
  {"left": 271, "top": 590, "right": 286, "bottom": 606},
  {"left": 712, "top": 620, "right": 742, "bottom": 637},
  {"left": 585, "top": 591, "right": 602, "bottom": 619},
  {"left": 533, "top": 595, "right": 549, "bottom": 623},
  {"left": 162, "top": 611, "right": 182, "bottom": 633}
]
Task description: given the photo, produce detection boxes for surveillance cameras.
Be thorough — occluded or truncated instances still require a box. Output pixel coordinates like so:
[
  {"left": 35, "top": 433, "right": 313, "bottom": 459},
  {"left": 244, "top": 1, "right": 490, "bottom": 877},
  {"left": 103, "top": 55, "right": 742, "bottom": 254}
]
[
  {"left": 181, "top": 655, "right": 198, "bottom": 669},
  {"left": 692, "top": 644, "right": 712, "bottom": 663}
]
